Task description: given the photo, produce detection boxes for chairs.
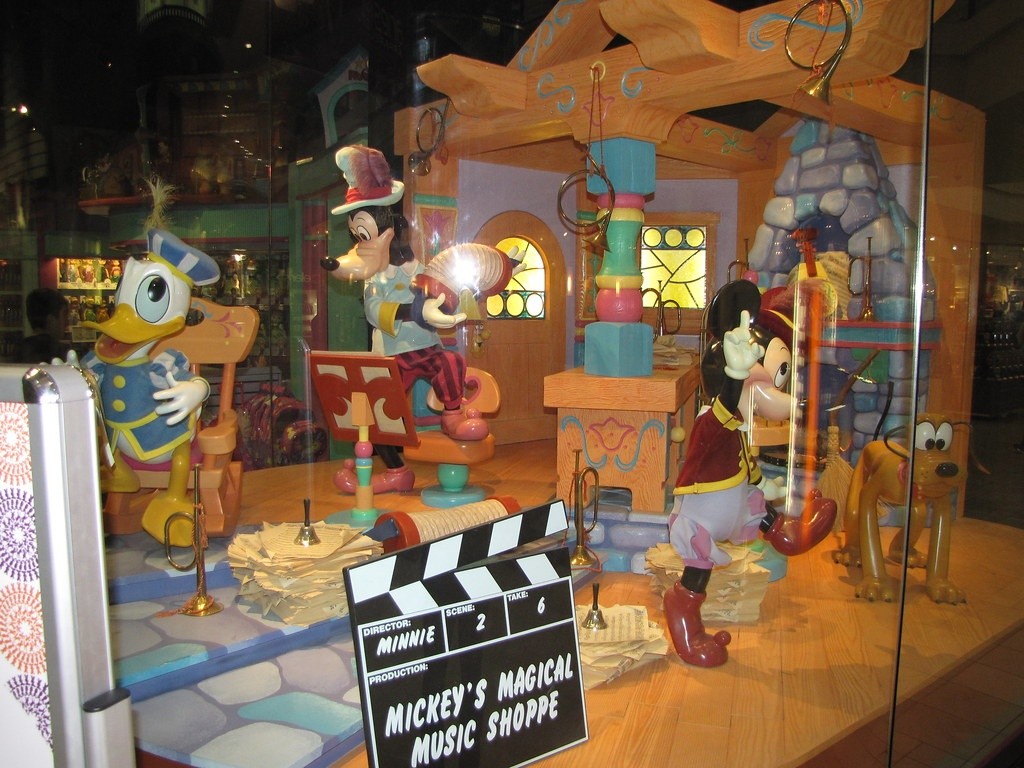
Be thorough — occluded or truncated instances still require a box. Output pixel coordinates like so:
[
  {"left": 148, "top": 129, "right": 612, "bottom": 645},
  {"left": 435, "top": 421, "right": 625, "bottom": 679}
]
[
  {"left": 405, "top": 368, "right": 502, "bottom": 509},
  {"left": 103, "top": 298, "right": 259, "bottom": 540}
]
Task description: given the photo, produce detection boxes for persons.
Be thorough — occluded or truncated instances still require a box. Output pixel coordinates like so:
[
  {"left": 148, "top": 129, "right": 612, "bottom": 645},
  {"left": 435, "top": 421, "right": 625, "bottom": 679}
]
[{"left": 11, "top": 287, "right": 73, "bottom": 405}]
